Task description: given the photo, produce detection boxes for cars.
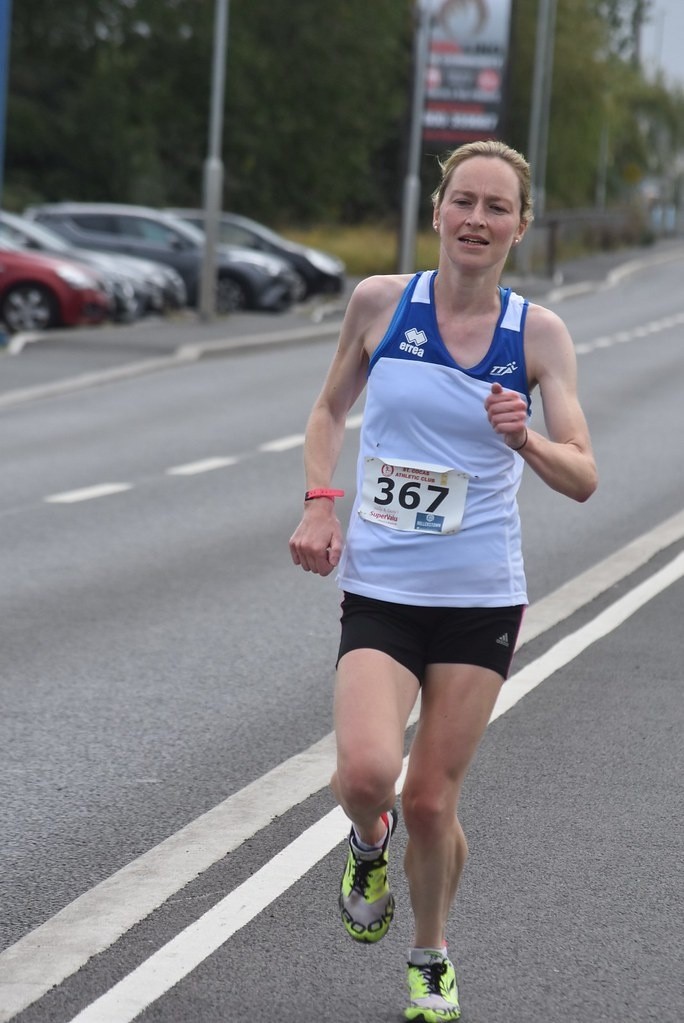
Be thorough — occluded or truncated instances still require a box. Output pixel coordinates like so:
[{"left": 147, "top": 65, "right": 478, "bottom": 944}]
[
  {"left": 0, "top": 237, "right": 122, "bottom": 335},
  {"left": 20, "top": 200, "right": 309, "bottom": 317},
  {"left": 164, "top": 205, "right": 347, "bottom": 301},
  {"left": 1, "top": 205, "right": 189, "bottom": 318}
]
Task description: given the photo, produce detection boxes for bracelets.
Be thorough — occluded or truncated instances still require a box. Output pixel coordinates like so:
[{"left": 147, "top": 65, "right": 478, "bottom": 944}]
[
  {"left": 304, "top": 488, "right": 344, "bottom": 502},
  {"left": 514, "top": 428, "right": 527, "bottom": 450}
]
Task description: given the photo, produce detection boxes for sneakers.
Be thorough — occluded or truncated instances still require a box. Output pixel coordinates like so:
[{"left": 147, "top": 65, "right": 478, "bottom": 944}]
[
  {"left": 338, "top": 808, "right": 398, "bottom": 944},
  {"left": 403, "top": 951, "right": 461, "bottom": 1023}
]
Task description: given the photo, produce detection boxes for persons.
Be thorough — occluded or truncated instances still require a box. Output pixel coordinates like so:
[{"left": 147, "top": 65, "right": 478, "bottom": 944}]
[{"left": 288, "top": 141, "right": 594, "bottom": 1023}]
